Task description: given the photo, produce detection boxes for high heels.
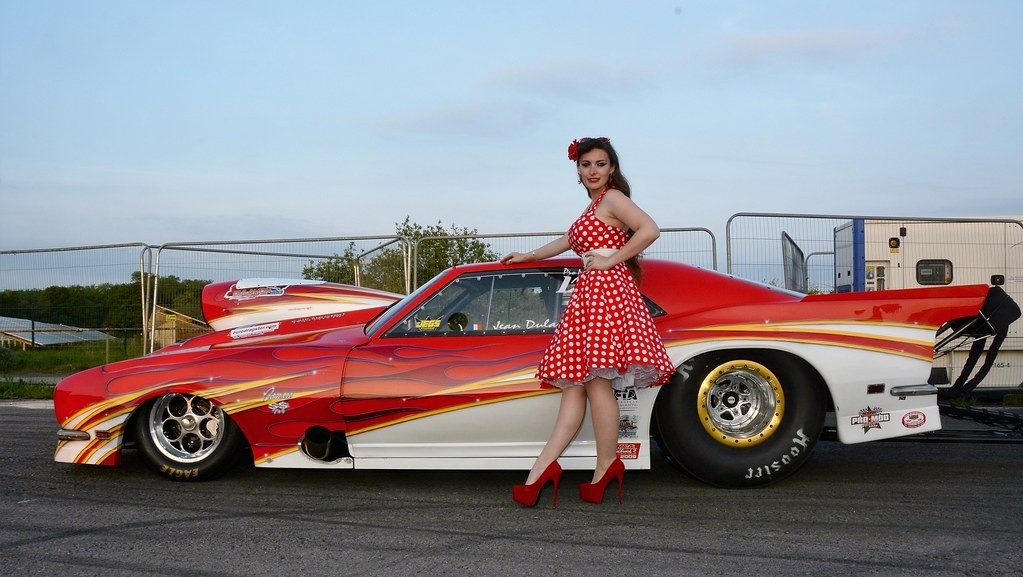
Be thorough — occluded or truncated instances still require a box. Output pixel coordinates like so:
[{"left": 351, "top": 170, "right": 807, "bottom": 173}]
[
  {"left": 578, "top": 454, "right": 625, "bottom": 504},
  {"left": 512, "top": 460, "right": 562, "bottom": 508}
]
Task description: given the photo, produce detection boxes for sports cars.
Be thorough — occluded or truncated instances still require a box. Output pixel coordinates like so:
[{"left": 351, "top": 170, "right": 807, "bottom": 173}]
[{"left": 54, "top": 257, "right": 991, "bottom": 491}]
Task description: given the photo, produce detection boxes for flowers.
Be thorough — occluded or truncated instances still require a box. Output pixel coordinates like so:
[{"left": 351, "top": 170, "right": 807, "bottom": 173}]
[{"left": 568, "top": 139, "right": 578, "bottom": 162}]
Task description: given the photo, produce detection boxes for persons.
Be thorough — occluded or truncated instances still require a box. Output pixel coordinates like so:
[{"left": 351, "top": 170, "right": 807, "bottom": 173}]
[{"left": 501, "top": 137, "right": 660, "bottom": 507}]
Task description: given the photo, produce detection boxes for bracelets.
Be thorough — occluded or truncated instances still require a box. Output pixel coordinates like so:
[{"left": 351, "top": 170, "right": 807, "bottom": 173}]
[{"left": 533, "top": 250, "right": 541, "bottom": 260}]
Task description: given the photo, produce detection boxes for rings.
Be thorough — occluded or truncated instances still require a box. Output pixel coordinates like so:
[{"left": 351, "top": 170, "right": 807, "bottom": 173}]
[{"left": 509, "top": 259, "right": 512, "bottom": 264}]
